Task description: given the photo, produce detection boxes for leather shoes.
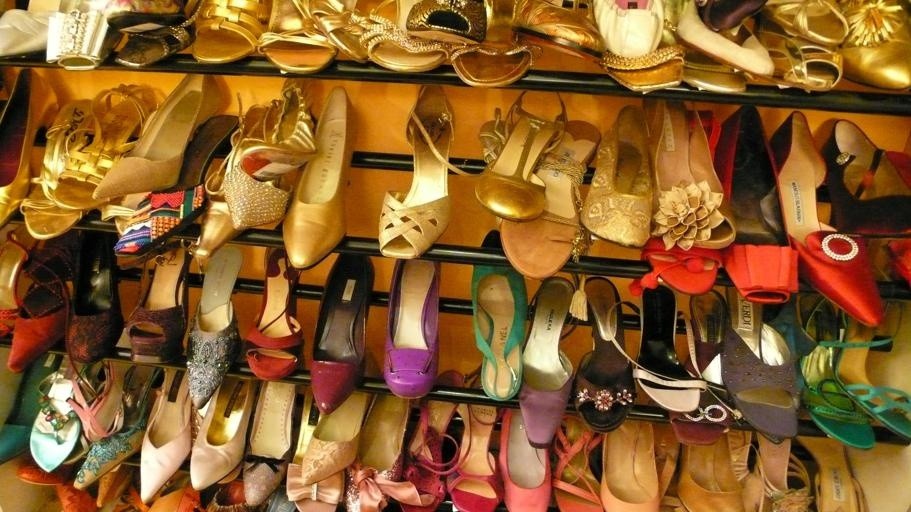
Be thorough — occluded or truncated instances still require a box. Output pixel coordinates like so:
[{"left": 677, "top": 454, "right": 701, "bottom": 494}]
[{"left": 0, "top": 344, "right": 865, "bottom": 512}]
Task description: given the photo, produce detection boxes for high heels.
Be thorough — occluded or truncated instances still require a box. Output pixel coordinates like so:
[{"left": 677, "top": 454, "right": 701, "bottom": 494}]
[
  {"left": 376, "top": 85, "right": 489, "bottom": 260},
  {"left": 283, "top": 87, "right": 350, "bottom": 270},
  {"left": 475, "top": 93, "right": 911, "bottom": 324}
]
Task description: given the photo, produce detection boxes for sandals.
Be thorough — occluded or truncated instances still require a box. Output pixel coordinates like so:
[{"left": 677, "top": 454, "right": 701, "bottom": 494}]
[
  {"left": 0, "top": 69, "right": 318, "bottom": 272},
  {"left": 0, "top": 1, "right": 911, "bottom": 93},
  {"left": 0, "top": 210, "right": 911, "bottom": 449}
]
